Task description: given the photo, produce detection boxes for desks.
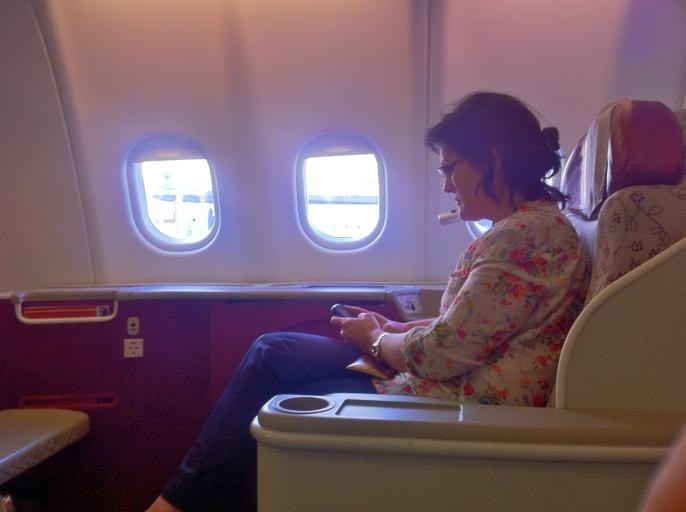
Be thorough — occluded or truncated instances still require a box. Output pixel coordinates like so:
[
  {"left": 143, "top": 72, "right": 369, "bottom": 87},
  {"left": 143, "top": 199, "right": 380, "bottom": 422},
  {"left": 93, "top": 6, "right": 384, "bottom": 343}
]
[{"left": 0, "top": 408, "right": 90, "bottom": 486}]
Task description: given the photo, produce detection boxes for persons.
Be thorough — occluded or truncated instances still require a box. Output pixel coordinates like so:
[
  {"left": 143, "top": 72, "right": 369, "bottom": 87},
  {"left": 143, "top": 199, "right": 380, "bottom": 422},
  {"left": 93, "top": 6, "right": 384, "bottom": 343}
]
[{"left": 146, "top": 91, "right": 591, "bottom": 512}]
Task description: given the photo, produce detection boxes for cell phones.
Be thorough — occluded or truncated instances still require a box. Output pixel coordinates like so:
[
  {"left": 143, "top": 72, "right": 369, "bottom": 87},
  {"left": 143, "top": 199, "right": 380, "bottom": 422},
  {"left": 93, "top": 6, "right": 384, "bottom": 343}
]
[{"left": 330, "top": 303, "right": 356, "bottom": 317}]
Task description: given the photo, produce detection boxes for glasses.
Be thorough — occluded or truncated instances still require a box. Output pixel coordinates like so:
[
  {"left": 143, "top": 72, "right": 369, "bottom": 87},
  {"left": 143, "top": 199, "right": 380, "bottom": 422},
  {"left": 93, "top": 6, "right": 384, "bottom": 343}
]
[{"left": 438, "top": 159, "right": 462, "bottom": 177}]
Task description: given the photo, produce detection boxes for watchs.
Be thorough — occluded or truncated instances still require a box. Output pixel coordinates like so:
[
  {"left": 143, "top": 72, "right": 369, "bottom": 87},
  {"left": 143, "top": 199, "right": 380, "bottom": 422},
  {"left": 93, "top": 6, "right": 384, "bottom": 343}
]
[{"left": 370, "top": 332, "right": 391, "bottom": 363}]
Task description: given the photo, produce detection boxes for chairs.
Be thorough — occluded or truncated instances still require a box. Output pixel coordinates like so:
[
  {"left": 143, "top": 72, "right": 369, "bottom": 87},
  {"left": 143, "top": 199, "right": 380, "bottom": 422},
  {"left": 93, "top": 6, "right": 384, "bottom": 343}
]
[{"left": 549, "top": 98, "right": 686, "bottom": 406}]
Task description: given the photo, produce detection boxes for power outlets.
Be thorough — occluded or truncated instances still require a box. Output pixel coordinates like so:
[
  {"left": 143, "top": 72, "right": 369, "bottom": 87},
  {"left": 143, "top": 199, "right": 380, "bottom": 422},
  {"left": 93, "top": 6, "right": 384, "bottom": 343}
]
[{"left": 124, "top": 339, "right": 143, "bottom": 358}]
